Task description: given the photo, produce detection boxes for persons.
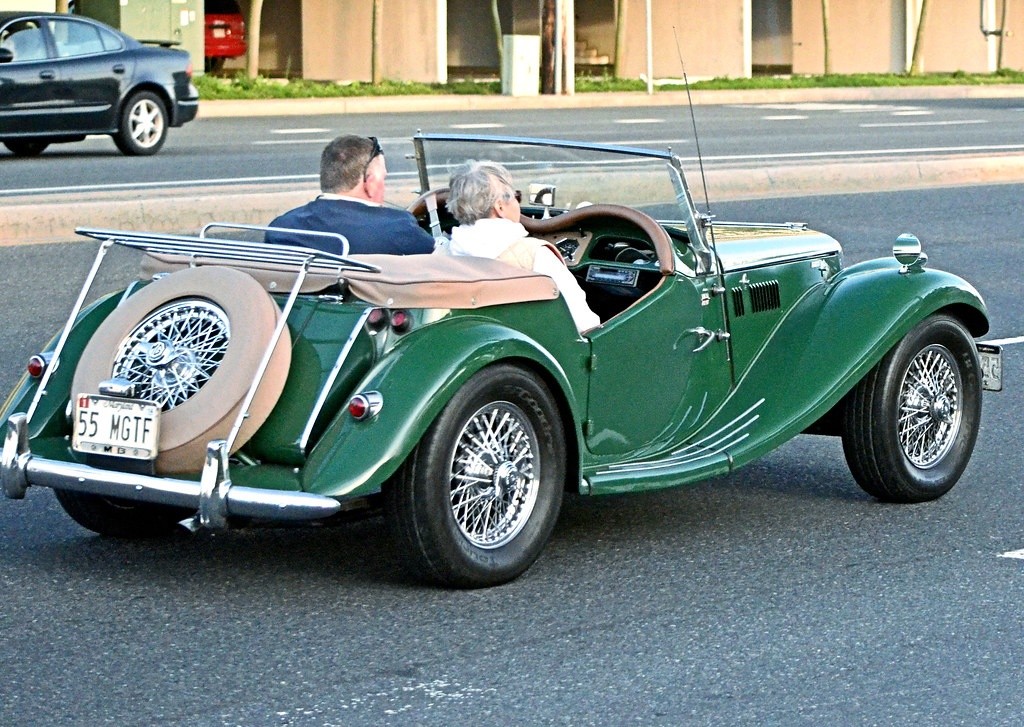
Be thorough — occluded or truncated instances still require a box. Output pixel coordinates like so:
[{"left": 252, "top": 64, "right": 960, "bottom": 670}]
[
  {"left": 262, "top": 134, "right": 435, "bottom": 254},
  {"left": 431, "top": 160, "right": 600, "bottom": 334}
]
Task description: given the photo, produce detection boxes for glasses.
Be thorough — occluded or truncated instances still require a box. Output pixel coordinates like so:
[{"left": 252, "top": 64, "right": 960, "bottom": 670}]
[
  {"left": 507, "top": 189, "right": 522, "bottom": 204},
  {"left": 361, "top": 135, "right": 381, "bottom": 183}
]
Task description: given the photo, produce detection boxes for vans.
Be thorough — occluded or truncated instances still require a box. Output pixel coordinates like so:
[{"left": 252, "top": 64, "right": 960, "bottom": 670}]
[{"left": 204, "top": 1, "right": 247, "bottom": 72}]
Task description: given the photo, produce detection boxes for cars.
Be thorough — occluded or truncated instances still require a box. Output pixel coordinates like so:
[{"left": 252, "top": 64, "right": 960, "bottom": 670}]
[
  {"left": 1, "top": 127, "right": 1003, "bottom": 592},
  {"left": 0, "top": 11, "right": 200, "bottom": 156}
]
